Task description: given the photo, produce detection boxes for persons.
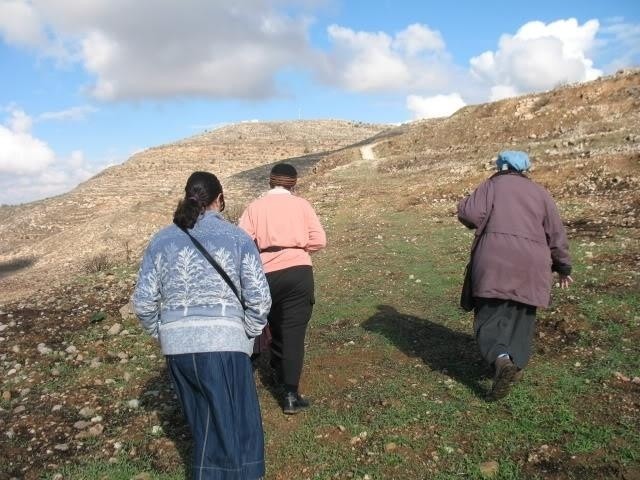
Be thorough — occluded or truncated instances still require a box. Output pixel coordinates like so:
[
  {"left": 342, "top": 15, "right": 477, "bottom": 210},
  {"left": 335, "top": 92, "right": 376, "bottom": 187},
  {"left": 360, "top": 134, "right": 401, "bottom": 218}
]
[
  {"left": 457, "top": 150, "right": 574, "bottom": 398},
  {"left": 237, "top": 164, "right": 327, "bottom": 414},
  {"left": 132, "top": 171, "right": 273, "bottom": 480}
]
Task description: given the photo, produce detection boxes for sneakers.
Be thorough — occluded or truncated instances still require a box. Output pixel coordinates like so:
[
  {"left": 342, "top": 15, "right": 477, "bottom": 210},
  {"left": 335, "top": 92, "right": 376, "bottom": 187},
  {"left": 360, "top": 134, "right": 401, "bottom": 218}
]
[
  {"left": 491, "top": 358, "right": 517, "bottom": 399},
  {"left": 284, "top": 396, "right": 310, "bottom": 413},
  {"left": 511, "top": 367, "right": 524, "bottom": 382}
]
